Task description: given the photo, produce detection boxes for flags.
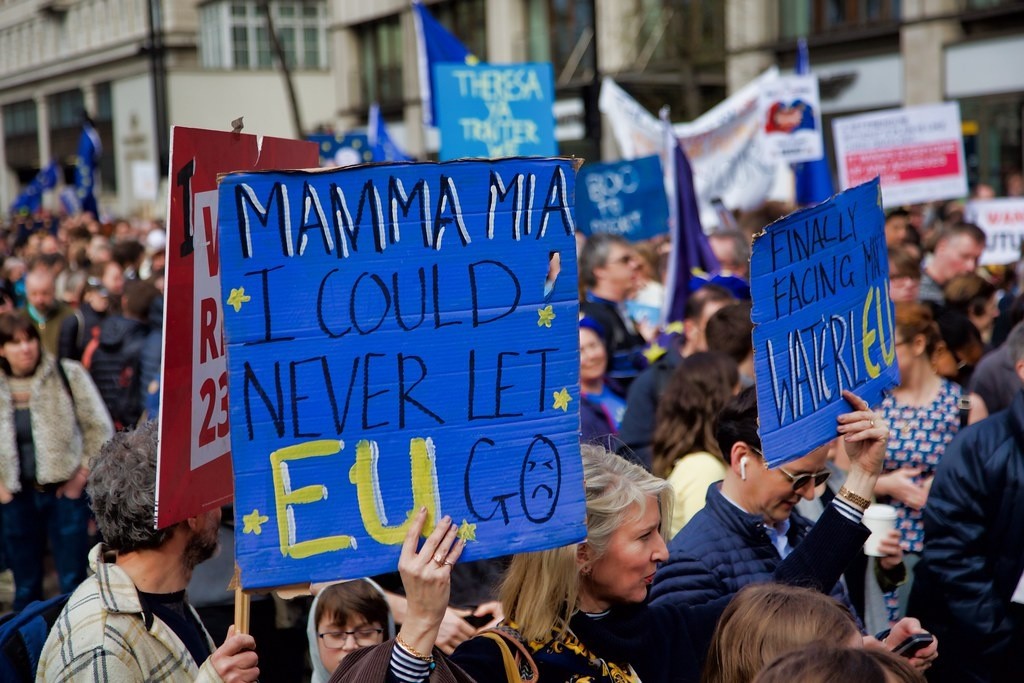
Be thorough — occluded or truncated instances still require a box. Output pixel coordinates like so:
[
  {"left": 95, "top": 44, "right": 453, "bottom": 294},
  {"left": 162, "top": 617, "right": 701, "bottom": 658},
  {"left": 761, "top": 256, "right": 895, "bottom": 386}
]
[
  {"left": 14, "top": 165, "right": 59, "bottom": 210},
  {"left": 413, "top": 2, "right": 482, "bottom": 128},
  {"left": 661, "top": 140, "right": 720, "bottom": 323},
  {"left": 70, "top": 124, "right": 102, "bottom": 210}
]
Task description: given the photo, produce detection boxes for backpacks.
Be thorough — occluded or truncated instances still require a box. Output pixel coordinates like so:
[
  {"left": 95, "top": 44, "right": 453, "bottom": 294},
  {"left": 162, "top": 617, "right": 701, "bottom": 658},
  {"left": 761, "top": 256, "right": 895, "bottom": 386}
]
[{"left": 0, "top": 592, "right": 73, "bottom": 683}]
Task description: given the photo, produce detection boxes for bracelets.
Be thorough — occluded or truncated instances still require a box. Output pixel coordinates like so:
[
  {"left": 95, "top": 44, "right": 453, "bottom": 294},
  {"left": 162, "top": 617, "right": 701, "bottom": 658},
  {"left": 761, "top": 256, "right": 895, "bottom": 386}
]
[
  {"left": 837, "top": 486, "right": 871, "bottom": 509},
  {"left": 396, "top": 632, "right": 435, "bottom": 669}
]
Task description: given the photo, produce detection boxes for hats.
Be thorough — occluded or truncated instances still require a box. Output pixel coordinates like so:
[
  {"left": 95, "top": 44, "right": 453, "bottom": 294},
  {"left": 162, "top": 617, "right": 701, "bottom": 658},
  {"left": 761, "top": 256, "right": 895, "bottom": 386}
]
[{"left": 579, "top": 310, "right": 605, "bottom": 338}]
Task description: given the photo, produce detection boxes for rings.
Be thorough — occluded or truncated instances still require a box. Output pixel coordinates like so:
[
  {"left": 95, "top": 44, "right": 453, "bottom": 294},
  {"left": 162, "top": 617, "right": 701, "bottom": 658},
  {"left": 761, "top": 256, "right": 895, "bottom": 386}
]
[
  {"left": 864, "top": 401, "right": 868, "bottom": 411},
  {"left": 443, "top": 560, "right": 453, "bottom": 570},
  {"left": 870, "top": 420, "right": 874, "bottom": 428},
  {"left": 433, "top": 555, "right": 444, "bottom": 566}
]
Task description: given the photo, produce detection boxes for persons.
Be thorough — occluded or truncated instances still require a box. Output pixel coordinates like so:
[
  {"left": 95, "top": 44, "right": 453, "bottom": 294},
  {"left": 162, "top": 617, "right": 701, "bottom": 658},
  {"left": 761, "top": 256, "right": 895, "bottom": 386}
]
[
  {"left": 0, "top": 204, "right": 1024, "bottom": 683},
  {"left": 367, "top": 112, "right": 412, "bottom": 163}
]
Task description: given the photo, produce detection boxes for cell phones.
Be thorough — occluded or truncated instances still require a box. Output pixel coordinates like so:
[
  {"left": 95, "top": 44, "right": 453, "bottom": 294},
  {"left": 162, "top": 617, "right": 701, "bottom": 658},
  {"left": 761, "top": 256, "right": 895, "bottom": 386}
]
[{"left": 890, "top": 634, "right": 934, "bottom": 659}]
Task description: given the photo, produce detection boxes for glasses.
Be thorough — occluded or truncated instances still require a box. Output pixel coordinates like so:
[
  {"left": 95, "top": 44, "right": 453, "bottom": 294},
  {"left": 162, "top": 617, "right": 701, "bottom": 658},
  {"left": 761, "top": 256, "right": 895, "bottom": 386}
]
[
  {"left": 749, "top": 445, "right": 831, "bottom": 490},
  {"left": 888, "top": 271, "right": 923, "bottom": 288},
  {"left": 318, "top": 629, "right": 383, "bottom": 649}
]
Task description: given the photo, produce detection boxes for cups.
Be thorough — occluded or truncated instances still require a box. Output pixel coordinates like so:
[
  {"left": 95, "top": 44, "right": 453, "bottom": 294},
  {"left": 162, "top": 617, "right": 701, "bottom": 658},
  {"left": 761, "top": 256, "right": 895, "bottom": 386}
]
[{"left": 862, "top": 503, "right": 896, "bottom": 557}]
